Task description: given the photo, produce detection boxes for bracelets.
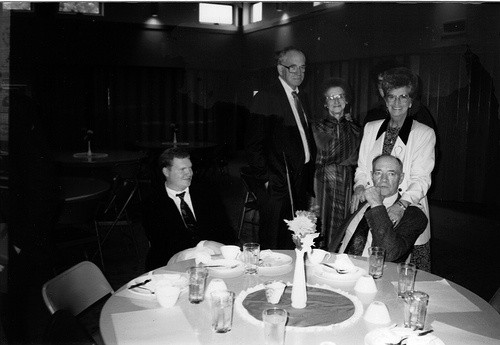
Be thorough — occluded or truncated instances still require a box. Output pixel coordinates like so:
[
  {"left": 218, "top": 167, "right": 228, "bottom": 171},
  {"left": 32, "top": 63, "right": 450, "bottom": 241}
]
[{"left": 396, "top": 201, "right": 407, "bottom": 211}]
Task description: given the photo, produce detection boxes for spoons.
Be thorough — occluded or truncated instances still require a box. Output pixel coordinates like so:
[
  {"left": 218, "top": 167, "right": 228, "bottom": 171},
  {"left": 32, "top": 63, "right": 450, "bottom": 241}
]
[
  {"left": 384, "top": 329, "right": 434, "bottom": 345},
  {"left": 322, "top": 262, "right": 348, "bottom": 274}
]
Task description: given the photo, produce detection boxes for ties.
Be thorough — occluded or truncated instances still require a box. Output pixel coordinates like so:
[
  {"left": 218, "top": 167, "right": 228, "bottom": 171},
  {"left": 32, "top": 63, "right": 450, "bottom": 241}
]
[
  {"left": 344, "top": 206, "right": 370, "bottom": 255},
  {"left": 292, "top": 91, "right": 312, "bottom": 160},
  {"left": 176, "top": 192, "right": 200, "bottom": 245}
]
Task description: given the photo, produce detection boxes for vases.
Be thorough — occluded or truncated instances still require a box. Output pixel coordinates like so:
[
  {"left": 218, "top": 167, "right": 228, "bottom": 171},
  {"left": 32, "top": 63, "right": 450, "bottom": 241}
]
[
  {"left": 291, "top": 248, "right": 307, "bottom": 309},
  {"left": 87, "top": 140, "right": 93, "bottom": 156},
  {"left": 173, "top": 132, "right": 177, "bottom": 143}
]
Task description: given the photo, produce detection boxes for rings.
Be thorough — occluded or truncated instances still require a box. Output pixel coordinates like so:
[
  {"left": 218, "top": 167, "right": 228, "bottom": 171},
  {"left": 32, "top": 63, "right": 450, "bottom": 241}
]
[{"left": 392, "top": 220, "right": 396, "bottom": 223}]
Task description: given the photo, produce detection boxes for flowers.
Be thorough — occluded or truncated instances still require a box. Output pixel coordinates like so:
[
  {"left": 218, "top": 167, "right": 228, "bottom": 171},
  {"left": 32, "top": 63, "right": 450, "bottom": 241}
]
[
  {"left": 81, "top": 128, "right": 94, "bottom": 141},
  {"left": 283, "top": 210, "right": 321, "bottom": 254},
  {"left": 171, "top": 123, "right": 180, "bottom": 132}
]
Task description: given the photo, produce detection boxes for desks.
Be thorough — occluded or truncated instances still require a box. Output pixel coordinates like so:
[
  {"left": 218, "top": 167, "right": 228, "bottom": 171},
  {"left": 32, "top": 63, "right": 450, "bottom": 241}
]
[
  {"left": 146, "top": 142, "right": 215, "bottom": 184},
  {"left": 58, "top": 149, "right": 141, "bottom": 210},
  {"left": 61, "top": 177, "right": 111, "bottom": 244},
  {"left": 99, "top": 249, "right": 500, "bottom": 345}
]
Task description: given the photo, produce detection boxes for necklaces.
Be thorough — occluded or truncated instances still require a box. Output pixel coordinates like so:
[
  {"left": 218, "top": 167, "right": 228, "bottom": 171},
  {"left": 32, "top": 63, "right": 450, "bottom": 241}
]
[{"left": 389, "top": 123, "right": 400, "bottom": 144}]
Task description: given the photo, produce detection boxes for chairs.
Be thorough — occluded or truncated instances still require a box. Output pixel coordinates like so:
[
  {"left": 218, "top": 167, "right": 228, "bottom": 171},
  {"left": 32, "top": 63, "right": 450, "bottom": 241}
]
[
  {"left": 86, "top": 169, "right": 138, "bottom": 270},
  {"left": 41, "top": 260, "right": 115, "bottom": 345}
]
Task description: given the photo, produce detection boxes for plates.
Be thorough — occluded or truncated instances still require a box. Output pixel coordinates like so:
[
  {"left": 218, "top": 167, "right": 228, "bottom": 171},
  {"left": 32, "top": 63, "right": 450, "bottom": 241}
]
[
  {"left": 206, "top": 260, "right": 243, "bottom": 274},
  {"left": 364, "top": 327, "right": 445, "bottom": 345},
  {"left": 127, "top": 274, "right": 190, "bottom": 297},
  {"left": 240, "top": 251, "right": 293, "bottom": 270},
  {"left": 314, "top": 264, "right": 367, "bottom": 282}
]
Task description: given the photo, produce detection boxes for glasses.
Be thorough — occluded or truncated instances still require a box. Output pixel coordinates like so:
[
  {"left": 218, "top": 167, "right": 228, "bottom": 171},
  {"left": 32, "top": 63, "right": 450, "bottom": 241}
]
[
  {"left": 280, "top": 64, "right": 307, "bottom": 73},
  {"left": 386, "top": 94, "right": 411, "bottom": 104},
  {"left": 325, "top": 94, "right": 346, "bottom": 103}
]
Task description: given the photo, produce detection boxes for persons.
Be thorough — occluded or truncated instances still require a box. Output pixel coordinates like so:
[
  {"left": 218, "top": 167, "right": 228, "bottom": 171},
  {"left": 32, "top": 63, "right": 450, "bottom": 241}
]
[
  {"left": 240, "top": 46, "right": 318, "bottom": 252},
  {"left": 141, "top": 147, "right": 237, "bottom": 265},
  {"left": 326, "top": 155, "right": 428, "bottom": 268},
  {"left": 310, "top": 78, "right": 363, "bottom": 257},
  {"left": 351, "top": 66, "right": 436, "bottom": 274}
]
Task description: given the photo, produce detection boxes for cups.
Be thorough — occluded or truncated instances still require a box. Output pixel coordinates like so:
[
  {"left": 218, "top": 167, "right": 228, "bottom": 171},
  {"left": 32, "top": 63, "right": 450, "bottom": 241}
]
[
  {"left": 403, "top": 290, "right": 429, "bottom": 330},
  {"left": 155, "top": 285, "right": 180, "bottom": 307},
  {"left": 209, "top": 290, "right": 234, "bottom": 333},
  {"left": 188, "top": 267, "right": 207, "bottom": 304},
  {"left": 368, "top": 247, "right": 385, "bottom": 279},
  {"left": 363, "top": 301, "right": 391, "bottom": 324},
  {"left": 205, "top": 278, "right": 228, "bottom": 299},
  {"left": 261, "top": 307, "right": 287, "bottom": 345},
  {"left": 353, "top": 275, "right": 376, "bottom": 293},
  {"left": 242, "top": 243, "right": 260, "bottom": 276},
  {"left": 397, "top": 263, "right": 416, "bottom": 298},
  {"left": 220, "top": 245, "right": 239, "bottom": 260},
  {"left": 263, "top": 279, "right": 287, "bottom": 304},
  {"left": 308, "top": 249, "right": 326, "bottom": 264}
]
